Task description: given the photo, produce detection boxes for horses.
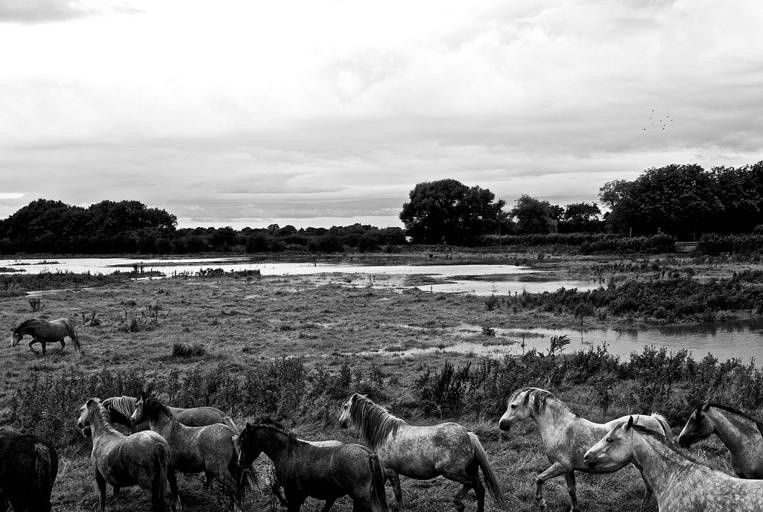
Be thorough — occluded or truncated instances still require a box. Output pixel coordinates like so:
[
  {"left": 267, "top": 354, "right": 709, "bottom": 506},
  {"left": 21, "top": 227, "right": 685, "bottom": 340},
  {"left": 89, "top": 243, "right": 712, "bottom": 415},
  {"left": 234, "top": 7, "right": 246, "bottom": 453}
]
[
  {"left": 497, "top": 383, "right": 674, "bottom": 512},
  {"left": 11, "top": 315, "right": 81, "bottom": 354},
  {"left": 71, "top": 387, "right": 507, "bottom": 512},
  {"left": 676, "top": 397, "right": 763, "bottom": 478},
  {"left": 0, "top": 422, "right": 62, "bottom": 512},
  {"left": 582, "top": 414, "right": 763, "bottom": 512}
]
